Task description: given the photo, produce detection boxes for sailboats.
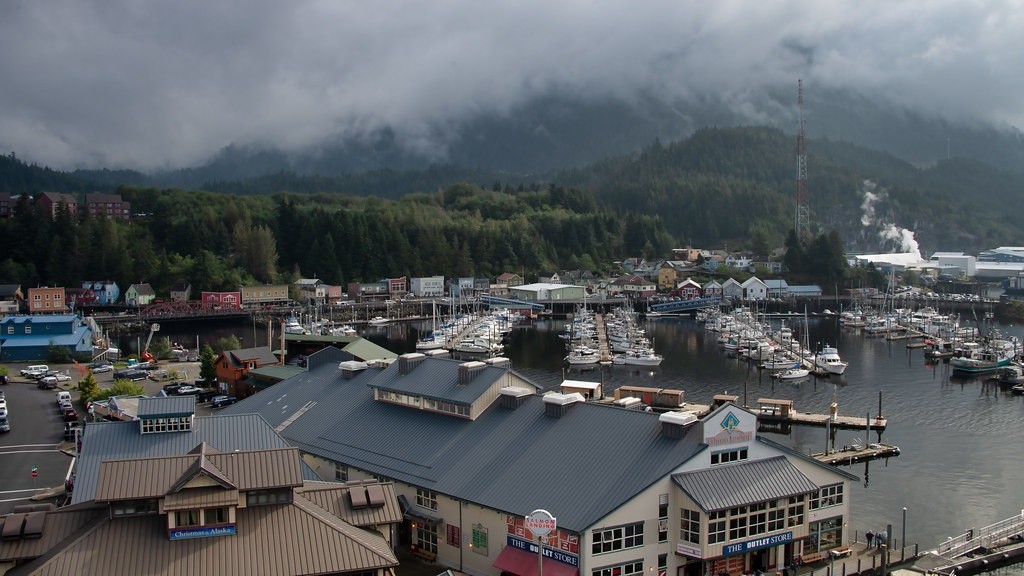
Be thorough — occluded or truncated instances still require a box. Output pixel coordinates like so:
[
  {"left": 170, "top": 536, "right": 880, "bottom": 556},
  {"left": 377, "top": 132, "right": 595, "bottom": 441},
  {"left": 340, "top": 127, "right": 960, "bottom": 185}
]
[
  {"left": 694, "top": 292, "right": 850, "bottom": 378},
  {"left": 415, "top": 285, "right": 477, "bottom": 350},
  {"left": 838, "top": 265, "right": 903, "bottom": 332}
]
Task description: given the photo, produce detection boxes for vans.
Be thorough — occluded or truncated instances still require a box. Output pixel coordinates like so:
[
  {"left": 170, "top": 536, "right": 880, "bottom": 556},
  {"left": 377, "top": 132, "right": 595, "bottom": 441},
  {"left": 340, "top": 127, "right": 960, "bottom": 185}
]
[
  {"left": 113, "top": 368, "right": 150, "bottom": 381},
  {"left": 905, "top": 291, "right": 981, "bottom": 301}
]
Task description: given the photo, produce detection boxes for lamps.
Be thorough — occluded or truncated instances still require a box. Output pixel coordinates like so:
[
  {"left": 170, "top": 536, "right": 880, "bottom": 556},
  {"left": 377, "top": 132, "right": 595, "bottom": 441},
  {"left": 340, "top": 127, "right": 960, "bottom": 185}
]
[
  {"left": 412, "top": 522, "right": 416, "bottom": 528},
  {"left": 844, "top": 523, "right": 846, "bottom": 527}
]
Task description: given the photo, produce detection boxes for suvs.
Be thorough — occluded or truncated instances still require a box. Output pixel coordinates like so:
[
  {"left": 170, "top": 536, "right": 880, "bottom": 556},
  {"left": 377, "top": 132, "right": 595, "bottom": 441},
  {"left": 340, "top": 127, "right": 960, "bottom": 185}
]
[
  {"left": 20, "top": 364, "right": 48, "bottom": 375},
  {"left": 37, "top": 376, "right": 58, "bottom": 389}
]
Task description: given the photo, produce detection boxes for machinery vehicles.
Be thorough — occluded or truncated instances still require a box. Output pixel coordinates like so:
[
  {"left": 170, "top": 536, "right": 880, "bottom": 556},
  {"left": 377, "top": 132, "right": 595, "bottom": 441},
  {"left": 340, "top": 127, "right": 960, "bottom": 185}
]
[{"left": 139, "top": 322, "right": 162, "bottom": 369}]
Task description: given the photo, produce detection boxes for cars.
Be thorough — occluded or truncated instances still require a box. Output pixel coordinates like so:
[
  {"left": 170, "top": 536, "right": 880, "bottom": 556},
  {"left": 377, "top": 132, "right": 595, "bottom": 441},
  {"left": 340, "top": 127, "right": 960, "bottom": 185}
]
[
  {"left": 164, "top": 381, "right": 238, "bottom": 407},
  {"left": 0, "top": 375, "right": 10, "bottom": 385},
  {"left": 57, "top": 391, "right": 80, "bottom": 440},
  {"left": 126, "top": 358, "right": 138, "bottom": 369},
  {"left": 85, "top": 360, "right": 114, "bottom": 374},
  {"left": 48, "top": 370, "right": 72, "bottom": 382},
  {"left": 895, "top": 285, "right": 913, "bottom": 293},
  {"left": 0, "top": 389, "right": 10, "bottom": 433}
]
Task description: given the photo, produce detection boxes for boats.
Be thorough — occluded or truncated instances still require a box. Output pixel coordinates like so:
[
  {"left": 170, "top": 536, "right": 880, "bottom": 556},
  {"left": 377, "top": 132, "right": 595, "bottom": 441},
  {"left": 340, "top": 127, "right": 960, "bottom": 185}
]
[
  {"left": 558, "top": 293, "right": 664, "bottom": 366},
  {"left": 457, "top": 307, "right": 521, "bottom": 353},
  {"left": 368, "top": 316, "right": 389, "bottom": 324},
  {"left": 286, "top": 309, "right": 358, "bottom": 338},
  {"left": 891, "top": 303, "right": 1023, "bottom": 392}
]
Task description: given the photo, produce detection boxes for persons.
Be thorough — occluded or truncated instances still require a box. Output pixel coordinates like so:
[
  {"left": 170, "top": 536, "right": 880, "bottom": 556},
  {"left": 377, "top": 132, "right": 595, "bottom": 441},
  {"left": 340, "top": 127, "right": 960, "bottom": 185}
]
[
  {"left": 783, "top": 566, "right": 789, "bottom": 576},
  {"left": 112, "top": 309, "right": 117, "bottom": 316},
  {"left": 55, "top": 497, "right": 63, "bottom": 508},
  {"left": 790, "top": 555, "right": 803, "bottom": 576},
  {"left": 789, "top": 371, "right": 791, "bottom": 374},
  {"left": 64, "top": 477, "right": 74, "bottom": 492},
  {"left": 76, "top": 314, "right": 82, "bottom": 327},
  {"left": 875, "top": 529, "right": 887, "bottom": 549},
  {"left": 142, "top": 302, "right": 284, "bottom": 317},
  {"left": 717, "top": 569, "right": 730, "bottom": 576},
  {"left": 866, "top": 530, "right": 874, "bottom": 549},
  {"left": 584, "top": 392, "right": 589, "bottom": 401}
]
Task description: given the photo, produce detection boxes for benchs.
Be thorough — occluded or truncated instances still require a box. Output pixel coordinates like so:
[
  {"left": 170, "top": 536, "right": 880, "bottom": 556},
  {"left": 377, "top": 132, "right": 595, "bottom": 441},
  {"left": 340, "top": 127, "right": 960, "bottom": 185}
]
[
  {"left": 801, "top": 553, "right": 825, "bottom": 569},
  {"left": 412, "top": 548, "right": 436, "bottom": 564},
  {"left": 829, "top": 546, "right": 853, "bottom": 560}
]
